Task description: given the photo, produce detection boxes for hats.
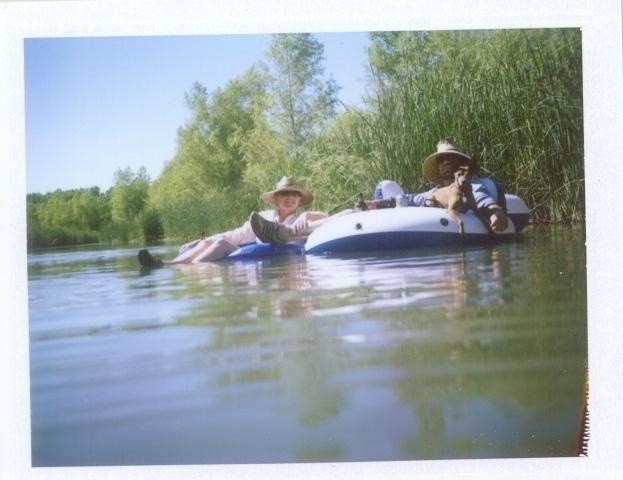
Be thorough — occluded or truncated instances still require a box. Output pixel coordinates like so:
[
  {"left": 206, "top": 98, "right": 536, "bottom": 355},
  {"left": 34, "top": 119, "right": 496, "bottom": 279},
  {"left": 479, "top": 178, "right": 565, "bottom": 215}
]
[
  {"left": 421, "top": 138, "right": 472, "bottom": 183},
  {"left": 261, "top": 176, "right": 314, "bottom": 207}
]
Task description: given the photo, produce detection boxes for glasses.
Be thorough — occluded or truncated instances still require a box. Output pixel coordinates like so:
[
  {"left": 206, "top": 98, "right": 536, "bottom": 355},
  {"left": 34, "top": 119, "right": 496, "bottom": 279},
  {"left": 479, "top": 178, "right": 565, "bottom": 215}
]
[
  {"left": 436, "top": 155, "right": 457, "bottom": 165},
  {"left": 277, "top": 191, "right": 301, "bottom": 197}
]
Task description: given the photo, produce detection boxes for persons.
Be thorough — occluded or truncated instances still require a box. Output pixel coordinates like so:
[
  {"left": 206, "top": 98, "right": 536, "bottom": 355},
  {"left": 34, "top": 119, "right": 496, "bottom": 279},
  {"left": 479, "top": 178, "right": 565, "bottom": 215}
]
[
  {"left": 250, "top": 140, "right": 509, "bottom": 247},
  {"left": 138, "top": 174, "right": 327, "bottom": 266}
]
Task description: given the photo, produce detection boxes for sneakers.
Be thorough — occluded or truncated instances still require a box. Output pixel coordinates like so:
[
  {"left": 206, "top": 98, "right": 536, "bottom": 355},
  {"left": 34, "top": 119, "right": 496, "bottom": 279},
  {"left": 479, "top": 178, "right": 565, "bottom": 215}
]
[
  {"left": 139, "top": 249, "right": 163, "bottom": 267},
  {"left": 250, "top": 211, "right": 289, "bottom": 246}
]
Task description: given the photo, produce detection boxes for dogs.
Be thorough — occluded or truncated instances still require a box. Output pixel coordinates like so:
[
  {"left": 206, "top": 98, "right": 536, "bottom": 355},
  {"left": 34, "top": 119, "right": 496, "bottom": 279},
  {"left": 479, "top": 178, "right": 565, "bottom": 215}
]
[{"left": 424, "top": 154, "right": 496, "bottom": 235}]
